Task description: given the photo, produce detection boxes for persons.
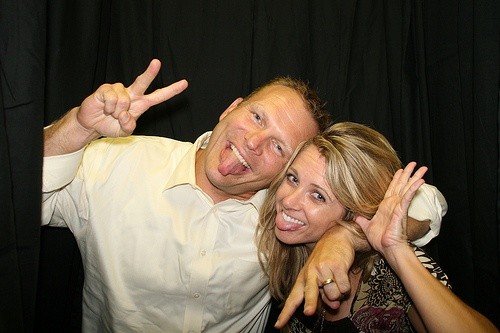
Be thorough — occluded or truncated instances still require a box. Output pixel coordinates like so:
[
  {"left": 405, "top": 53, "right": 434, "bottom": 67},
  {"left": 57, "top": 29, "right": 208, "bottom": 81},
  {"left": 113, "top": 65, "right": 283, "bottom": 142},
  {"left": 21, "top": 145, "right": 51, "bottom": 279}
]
[
  {"left": 255, "top": 122, "right": 500, "bottom": 333},
  {"left": 39, "top": 59, "right": 332, "bottom": 333}
]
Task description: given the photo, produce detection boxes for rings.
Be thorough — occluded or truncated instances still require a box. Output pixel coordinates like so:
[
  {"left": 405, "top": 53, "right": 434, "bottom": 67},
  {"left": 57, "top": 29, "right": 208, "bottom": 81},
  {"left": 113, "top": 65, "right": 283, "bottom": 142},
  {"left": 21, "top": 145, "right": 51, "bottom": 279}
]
[{"left": 322, "top": 278, "right": 334, "bottom": 286}]
